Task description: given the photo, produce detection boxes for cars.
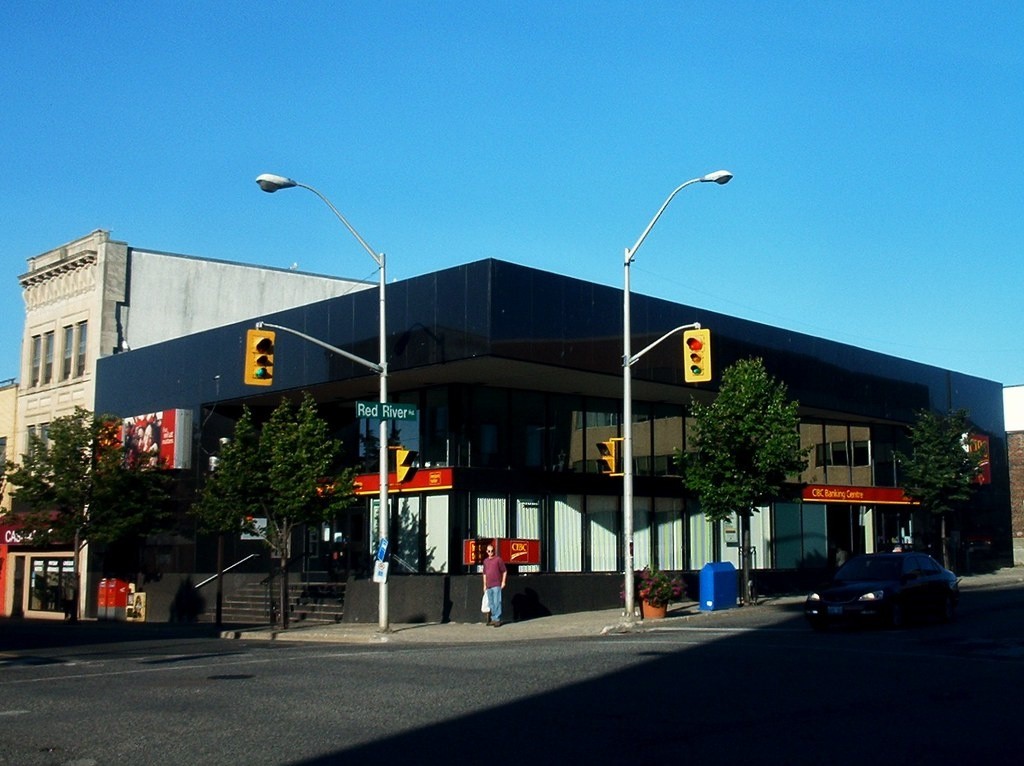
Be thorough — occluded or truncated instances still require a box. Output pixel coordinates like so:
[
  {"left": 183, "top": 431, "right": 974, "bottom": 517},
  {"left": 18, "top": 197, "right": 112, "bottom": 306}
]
[{"left": 804, "top": 553, "right": 960, "bottom": 631}]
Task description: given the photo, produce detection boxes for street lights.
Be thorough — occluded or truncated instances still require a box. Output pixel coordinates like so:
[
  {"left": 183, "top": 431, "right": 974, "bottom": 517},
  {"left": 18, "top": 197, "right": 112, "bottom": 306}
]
[
  {"left": 621, "top": 168, "right": 734, "bottom": 620},
  {"left": 256, "top": 175, "right": 388, "bottom": 633}
]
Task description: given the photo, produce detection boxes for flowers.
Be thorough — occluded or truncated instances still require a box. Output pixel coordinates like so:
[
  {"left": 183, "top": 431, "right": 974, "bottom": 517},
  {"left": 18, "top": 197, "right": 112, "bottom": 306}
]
[{"left": 618, "top": 566, "right": 689, "bottom": 609}]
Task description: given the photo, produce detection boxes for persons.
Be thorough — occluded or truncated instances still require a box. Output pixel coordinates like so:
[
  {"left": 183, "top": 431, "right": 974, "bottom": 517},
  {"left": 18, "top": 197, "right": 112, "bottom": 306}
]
[
  {"left": 836, "top": 548, "right": 847, "bottom": 567},
  {"left": 483, "top": 545, "right": 507, "bottom": 627},
  {"left": 124, "top": 423, "right": 159, "bottom": 466}
]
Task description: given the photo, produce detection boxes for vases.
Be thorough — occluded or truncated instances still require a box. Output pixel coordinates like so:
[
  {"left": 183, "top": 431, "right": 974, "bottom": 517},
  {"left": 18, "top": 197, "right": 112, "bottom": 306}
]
[{"left": 638, "top": 599, "right": 667, "bottom": 618}]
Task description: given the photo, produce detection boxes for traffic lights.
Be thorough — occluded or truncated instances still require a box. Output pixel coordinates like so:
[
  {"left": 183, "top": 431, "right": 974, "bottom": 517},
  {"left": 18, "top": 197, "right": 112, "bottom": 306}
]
[
  {"left": 682, "top": 329, "right": 712, "bottom": 383},
  {"left": 395, "top": 449, "right": 420, "bottom": 483},
  {"left": 596, "top": 441, "right": 617, "bottom": 473},
  {"left": 245, "top": 330, "right": 276, "bottom": 387}
]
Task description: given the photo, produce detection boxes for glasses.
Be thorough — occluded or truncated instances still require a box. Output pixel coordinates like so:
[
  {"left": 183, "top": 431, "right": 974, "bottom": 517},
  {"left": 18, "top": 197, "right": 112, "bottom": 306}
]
[{"left": 488, "top": 550, "right": 494, "bottom": 553}]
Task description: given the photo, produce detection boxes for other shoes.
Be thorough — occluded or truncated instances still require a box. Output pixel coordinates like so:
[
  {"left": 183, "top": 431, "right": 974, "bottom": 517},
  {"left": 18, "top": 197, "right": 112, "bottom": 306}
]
[
  {"left": 495, "top": 621, "right": 501, "bottom": 627},
  {"left": 487, "top": 621, "right": 496, "bottom": 625}
]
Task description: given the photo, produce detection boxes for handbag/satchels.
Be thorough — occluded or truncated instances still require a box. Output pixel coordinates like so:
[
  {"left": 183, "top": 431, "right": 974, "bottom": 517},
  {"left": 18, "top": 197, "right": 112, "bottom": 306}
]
[{"left": 480, "top": 590, "right": 490, "bottom": 612}]
